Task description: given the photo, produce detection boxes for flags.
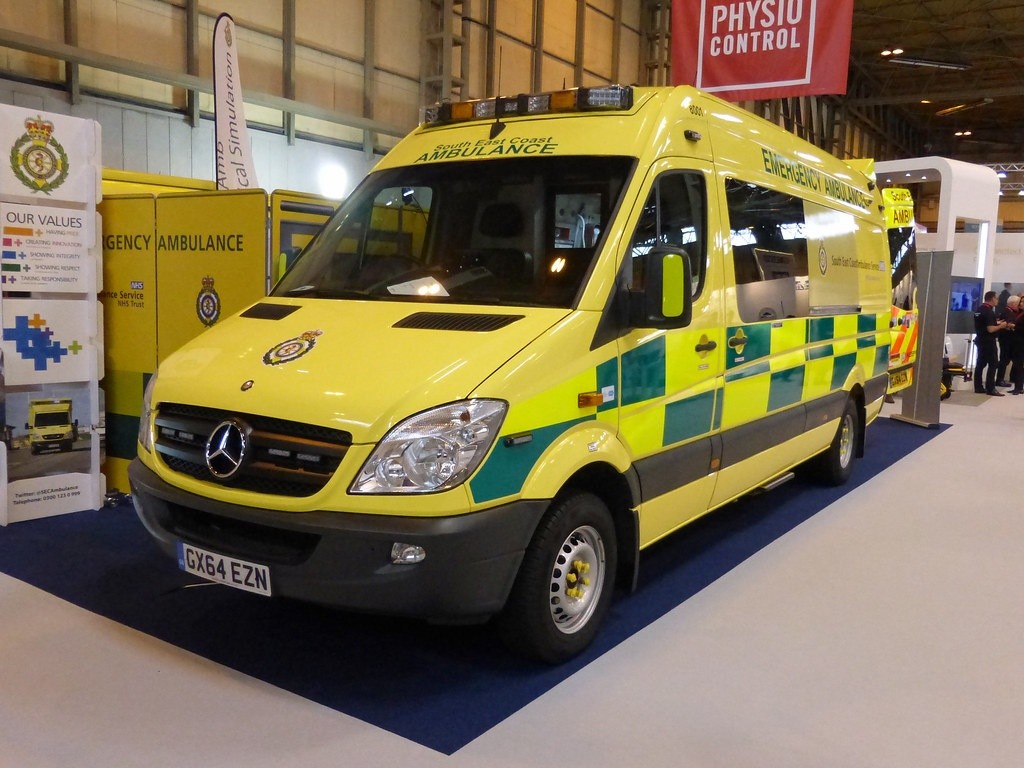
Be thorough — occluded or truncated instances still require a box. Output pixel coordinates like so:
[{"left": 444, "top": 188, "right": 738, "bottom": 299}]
[{"left": 669, "top": 0, "right": 854, "bottom": 101}]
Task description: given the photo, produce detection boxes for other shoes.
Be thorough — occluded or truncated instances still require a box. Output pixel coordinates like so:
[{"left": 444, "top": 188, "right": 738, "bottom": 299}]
[
  {"left": 975, "top": 388, "right": 986, "bottom": 393},
  {"left": 1007, "top": 390, "right": 1024, "bottom": 395},
  {"left": 996, "top": 381, "right": 1012, "bottom": 387},
  {"left": 987, "top": 390, "right": 1006, "bottom": 396}
]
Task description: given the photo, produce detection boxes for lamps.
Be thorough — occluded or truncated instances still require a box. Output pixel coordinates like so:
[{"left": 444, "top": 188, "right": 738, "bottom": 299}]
[
  {"left": 937, "top": 98, "right": 994, "bottom": 118},
  {"left": 889, "top": 57, "right": 973, "bottom": 72}
]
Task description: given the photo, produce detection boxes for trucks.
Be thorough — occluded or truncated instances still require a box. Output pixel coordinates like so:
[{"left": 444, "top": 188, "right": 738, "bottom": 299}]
[
  {"left": 24, "top": 398, "right": 79, "bottom": 455},
  {"left": 103, "top": 165, "right": 433, "bottom": 501}
]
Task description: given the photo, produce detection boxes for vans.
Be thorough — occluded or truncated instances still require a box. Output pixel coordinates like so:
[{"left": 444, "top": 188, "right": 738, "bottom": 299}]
[
  {"left": 879, "top": 187, "right": 919, "bottom": 402},
  {"left": 123, "top": 82, "right": 893, "bottom": 663}
]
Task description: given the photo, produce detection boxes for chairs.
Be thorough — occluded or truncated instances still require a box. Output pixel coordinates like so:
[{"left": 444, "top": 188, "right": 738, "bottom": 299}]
[
  {"left": 469, "top": 247, "right": 535, "bottom": 300},
  {"left": 537, "top": 255, "right": 580, "bottom": 309}
]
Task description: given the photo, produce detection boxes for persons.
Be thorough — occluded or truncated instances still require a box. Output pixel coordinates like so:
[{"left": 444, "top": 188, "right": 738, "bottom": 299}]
[
  {"left": 995, "top": 295, "right": 1024, "bottom": 395},
  {"left": 973, "top": 291, "right": 1007, "bottom": 397},
  {"left": 997, "top": 283, "right": 1012, "bottom": 314}
]
